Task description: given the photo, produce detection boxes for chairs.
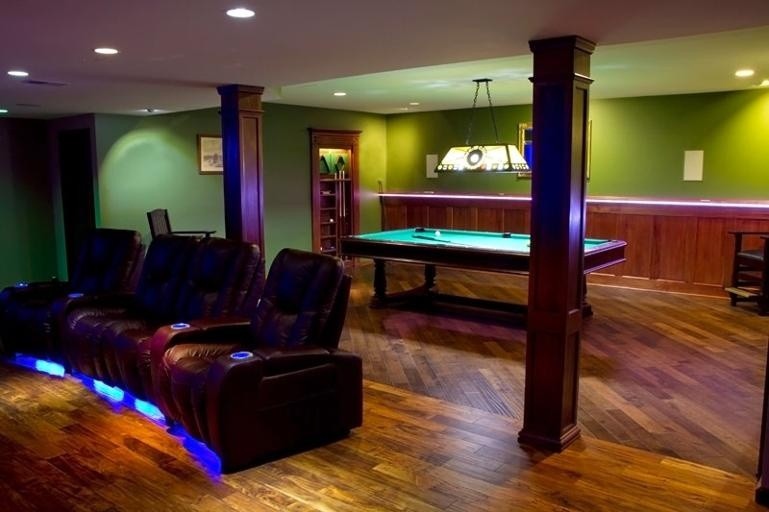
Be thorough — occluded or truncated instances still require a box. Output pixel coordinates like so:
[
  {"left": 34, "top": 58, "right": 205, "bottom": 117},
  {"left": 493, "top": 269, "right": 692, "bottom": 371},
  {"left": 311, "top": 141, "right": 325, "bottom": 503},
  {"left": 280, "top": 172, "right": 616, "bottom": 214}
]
[
  {"left": 726, "top": 227, "right": 769, "bottom": 315},
  {"left": 147, "top": 208, "right": 215, "bottom": 237}
]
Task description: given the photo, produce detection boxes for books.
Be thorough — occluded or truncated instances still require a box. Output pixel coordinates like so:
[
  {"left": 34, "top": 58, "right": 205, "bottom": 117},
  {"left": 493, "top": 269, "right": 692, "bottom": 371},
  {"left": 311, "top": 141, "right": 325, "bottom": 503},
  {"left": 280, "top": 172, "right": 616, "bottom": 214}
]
[{"left": 725, "top": 285, "right": 764, "bottom": 299}]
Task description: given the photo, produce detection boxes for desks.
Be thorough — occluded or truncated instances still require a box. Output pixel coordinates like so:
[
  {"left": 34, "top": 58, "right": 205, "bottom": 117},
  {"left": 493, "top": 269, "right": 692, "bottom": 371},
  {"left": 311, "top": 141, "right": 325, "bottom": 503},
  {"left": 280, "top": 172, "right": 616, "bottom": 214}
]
[{"left": 337, "top": 225, "right": 628, "bottom": 319}]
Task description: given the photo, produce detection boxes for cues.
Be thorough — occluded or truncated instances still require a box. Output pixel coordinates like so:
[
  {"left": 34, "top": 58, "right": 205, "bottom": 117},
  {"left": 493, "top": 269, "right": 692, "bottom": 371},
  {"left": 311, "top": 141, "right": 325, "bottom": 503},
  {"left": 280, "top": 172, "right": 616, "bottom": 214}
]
[{"left": 413, "top": 236, "right": 472, "bottom": 246}]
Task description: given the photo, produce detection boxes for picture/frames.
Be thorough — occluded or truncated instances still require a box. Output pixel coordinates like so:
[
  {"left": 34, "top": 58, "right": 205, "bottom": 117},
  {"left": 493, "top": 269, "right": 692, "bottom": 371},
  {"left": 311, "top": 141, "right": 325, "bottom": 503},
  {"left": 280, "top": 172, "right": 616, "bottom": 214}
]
[
  {"left": 518, "top": 118, "right": 594, "bottom": 182},
  {"left": 319, "top": 148, "right": 352, "bottom": 176},
  {"left": 197, "top": 133, "right": 224, "bottom": 175}
]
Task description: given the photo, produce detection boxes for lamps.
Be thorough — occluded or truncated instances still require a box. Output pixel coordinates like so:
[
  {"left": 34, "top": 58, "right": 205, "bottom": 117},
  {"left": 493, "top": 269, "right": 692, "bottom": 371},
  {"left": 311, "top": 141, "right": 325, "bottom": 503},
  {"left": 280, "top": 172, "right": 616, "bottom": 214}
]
[{"left": 433, "top": 79, "right": 533, "bottom": 173}]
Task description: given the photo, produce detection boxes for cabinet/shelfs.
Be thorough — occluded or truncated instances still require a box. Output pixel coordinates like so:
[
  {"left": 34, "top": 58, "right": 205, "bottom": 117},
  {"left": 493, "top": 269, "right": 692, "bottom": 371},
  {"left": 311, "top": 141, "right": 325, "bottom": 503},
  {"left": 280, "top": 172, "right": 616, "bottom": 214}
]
[{"left": 317, "top": 171, "right": 354, "bottom": 267}]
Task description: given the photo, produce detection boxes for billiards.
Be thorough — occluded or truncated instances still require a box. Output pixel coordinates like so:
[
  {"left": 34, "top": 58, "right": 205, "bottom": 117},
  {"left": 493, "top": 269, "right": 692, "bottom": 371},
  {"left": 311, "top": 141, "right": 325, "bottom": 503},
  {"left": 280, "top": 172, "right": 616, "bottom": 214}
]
[
  {"left": 527, "top": 240, "right": 530, "bottom": 246},
  {"left": 435, "top": 231, "right": 440, "bottom": 236}
]
